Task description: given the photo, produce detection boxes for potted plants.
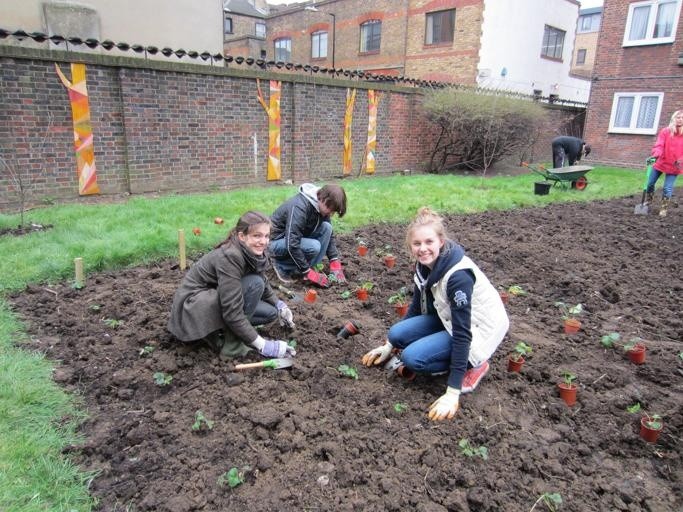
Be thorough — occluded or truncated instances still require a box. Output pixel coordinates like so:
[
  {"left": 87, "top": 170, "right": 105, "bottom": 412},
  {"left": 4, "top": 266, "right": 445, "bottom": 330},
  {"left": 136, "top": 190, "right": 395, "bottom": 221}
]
[
  {"left": 507, "top": 341, "right": 531, "bottom": 374},
  {"left": 388, "top": 285, "right": 412, "bottom": 315},
  {"left": 496, "top": 284, "right": 526, "bottom": 305},
  {"left": 338, "top": 320, "right": 361, "bottom": 339},
  {"left": 628, "top": 402, "right": 663, "bottom": 440},
  {"left": 340, "top": 282, "right": 374, "bottom": 299},
  {"left": 554, "top": 299, "right": 583, "bottom": 333},
  {"left": 378, "top": 244, "right": 395, "bottom": 265},
  {"left": 601, "top": 330, "right": 646, "bottom": 366},
  {"left": 556, "top": 371, "right": 577, "bottom": 402},
  {"left": 356, "top": 236, "right": 368, "bottom": 255},
  {"left": 312, "top": 264, "right": 339, "bottom": 288}
]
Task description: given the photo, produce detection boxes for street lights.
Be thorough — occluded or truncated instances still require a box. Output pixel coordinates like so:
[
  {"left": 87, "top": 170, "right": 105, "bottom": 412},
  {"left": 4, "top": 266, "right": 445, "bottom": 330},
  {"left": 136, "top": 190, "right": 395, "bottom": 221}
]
[{"left": 303, "top": 5, "right": 335, "bottom": 68}]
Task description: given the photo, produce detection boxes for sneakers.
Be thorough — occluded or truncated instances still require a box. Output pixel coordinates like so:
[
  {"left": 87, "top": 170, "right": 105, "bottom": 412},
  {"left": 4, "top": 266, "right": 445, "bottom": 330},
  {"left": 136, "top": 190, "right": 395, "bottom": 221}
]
[
  {"left": 459, "top": 360, "right": 491, "bottom": 395},
  {"left": 267, "top": 258, "right": 297, "bottom": 284}
]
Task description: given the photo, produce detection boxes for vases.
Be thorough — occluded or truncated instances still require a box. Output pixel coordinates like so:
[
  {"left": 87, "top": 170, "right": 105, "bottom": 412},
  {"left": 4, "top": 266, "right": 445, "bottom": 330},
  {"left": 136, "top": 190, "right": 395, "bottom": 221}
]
[
  {"left": 304, "top": 289, "right": 316, "bottom": 303},
  {"left": 192, "top": 229, "right": 200, "bottom": 235},
  {"left": 398, "top": 364, "right": 417, "bottom": 381},
  {"left": 215, "top": 217, "right": 223, "bottom": 225}
]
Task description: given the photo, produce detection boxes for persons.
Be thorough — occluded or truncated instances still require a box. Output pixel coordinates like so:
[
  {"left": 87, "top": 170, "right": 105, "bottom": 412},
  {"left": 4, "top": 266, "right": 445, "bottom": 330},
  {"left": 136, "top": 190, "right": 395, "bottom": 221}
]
[
  {"left": 551, "top": 135, "right": 592, "bottom": 189},
  {"left": 362, "top": 205, "right": 510, "bottom": 423},
  {"left": 644, "top": 110, "right": 683, "bottom": 219},
  {"left": 267, "top": 183, "right": 349, "bottom": 290},
  {"left": 166, "top": 211, "right": 297, "bottom": 362}
]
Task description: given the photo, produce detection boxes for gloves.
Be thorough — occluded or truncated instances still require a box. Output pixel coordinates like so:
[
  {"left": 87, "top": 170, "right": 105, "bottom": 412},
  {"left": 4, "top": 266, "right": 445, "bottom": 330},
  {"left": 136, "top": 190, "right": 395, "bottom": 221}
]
[
  {"left": 325, "top": 258, "right": 349, "bottom": 284},
  {"left": 672, "top": 157, "right": 683, "bottom": 172},
  {"left": 255, "top": 338, "right": 297, "bottom": 361},
  {"left": 272, "top": 299, "right": 296, "bottom": 329},
  {"left": 306, "top": 268, "right": 330, "bottom": 290},
  {"left": 360, "top": 341, "right": 394, "bottom": 369},
  {"left": 644, "top": 154, "right": 661, "bottom": 166},
  {"left": 428, "top": 387, "right": 461, "bottom": 423}
]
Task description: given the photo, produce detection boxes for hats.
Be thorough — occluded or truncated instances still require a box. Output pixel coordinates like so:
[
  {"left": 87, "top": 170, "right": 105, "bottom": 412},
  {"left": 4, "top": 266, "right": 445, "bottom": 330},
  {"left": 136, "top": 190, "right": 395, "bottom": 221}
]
[{"left": 583, "top": 145, "right": 593, "bottom": 159}]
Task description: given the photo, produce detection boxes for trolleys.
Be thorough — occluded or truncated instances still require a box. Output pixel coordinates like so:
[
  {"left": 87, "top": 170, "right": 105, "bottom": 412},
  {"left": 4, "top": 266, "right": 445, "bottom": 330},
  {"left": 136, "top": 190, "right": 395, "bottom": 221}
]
[{"left": 519, "top": 160, "right": 594, "bottom": 190}]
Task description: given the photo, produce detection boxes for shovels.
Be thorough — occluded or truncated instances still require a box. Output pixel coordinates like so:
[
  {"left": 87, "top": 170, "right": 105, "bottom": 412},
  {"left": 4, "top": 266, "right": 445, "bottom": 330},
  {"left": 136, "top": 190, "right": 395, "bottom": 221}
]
[
  {"left": 235, "top": 358, "right": 295, "bottom": 370},
  {"left": 634, "top": 159, "right": 656, "bottom": 215}
]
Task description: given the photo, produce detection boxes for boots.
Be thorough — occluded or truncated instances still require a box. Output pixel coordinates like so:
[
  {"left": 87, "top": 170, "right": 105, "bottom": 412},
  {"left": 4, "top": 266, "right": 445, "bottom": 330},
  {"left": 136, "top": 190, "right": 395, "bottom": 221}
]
[
  {"left": 641, "top": 190, "right": 655, "bottom": 213},
  {"left": 659, "top": 195, "right": 671, "bottom": 221},
  {"left": 217, "top": 329, "right": 255, "bottom": 361}
]
